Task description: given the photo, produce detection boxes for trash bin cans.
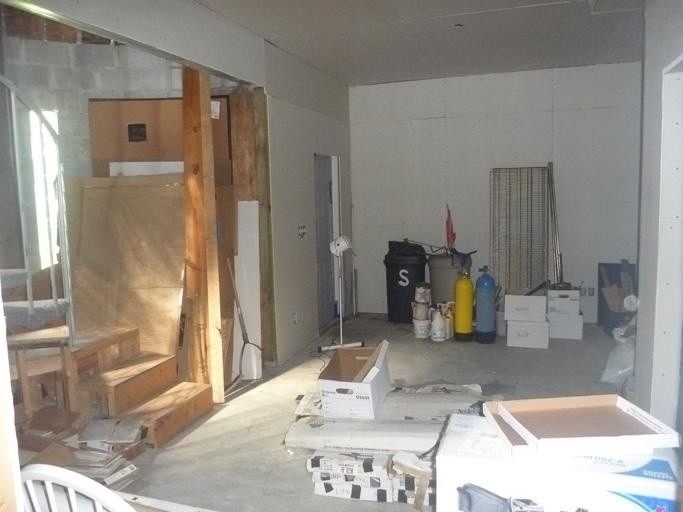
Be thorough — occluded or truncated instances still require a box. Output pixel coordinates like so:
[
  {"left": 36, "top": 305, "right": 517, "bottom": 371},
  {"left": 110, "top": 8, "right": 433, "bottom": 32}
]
[
  {"left": 384, "top": 241, "right": 427, "bottom": 324},
  {"left": 428, "top": 254, "right": 472, "bottom": 306}
]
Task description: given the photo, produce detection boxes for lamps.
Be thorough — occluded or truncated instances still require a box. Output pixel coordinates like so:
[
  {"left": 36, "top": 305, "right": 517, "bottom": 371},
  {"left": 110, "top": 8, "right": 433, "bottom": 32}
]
[{"left": 317, "top": 235, "right": 364, "bottom": 352}]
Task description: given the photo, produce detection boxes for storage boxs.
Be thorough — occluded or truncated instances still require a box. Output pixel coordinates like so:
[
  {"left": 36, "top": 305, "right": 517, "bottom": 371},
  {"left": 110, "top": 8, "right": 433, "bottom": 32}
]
[
  {"left": 547, "top": 290, "right": 580, "bottom": 315},
  {"left": 317, "top": 348, "right": 391, "bottom": 420},
  {"left": 504, "top": 287, "right": 547, "bottom": 322},
  {"left": 507, "top": 321, "right": 549, "bottom": 349},
  {"left": 548, "top": 310, "right": 583, "bottom": 340}
]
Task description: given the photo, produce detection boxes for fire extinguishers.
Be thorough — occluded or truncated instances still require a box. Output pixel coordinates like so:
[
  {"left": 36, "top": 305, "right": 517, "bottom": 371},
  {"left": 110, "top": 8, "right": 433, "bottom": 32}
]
[
  {"left": 475, "top": 265, "right": 498, "bottom": 343},
  {"left": 453, "top": 269, "right": 473, "bottom": 341}
]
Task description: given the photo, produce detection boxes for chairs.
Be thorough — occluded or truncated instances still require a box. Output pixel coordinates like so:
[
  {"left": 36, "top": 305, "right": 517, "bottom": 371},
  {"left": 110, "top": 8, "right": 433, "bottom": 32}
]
[{"left": 21, "top": 465, "right": 133, "bottom": 512}]
